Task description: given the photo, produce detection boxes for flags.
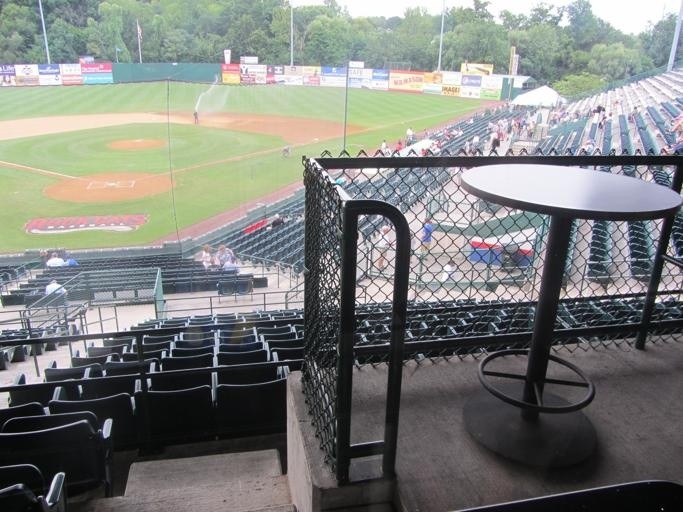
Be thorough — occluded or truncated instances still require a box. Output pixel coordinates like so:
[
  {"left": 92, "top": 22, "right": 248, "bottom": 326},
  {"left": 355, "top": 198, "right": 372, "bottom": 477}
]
[{"left": 138, "top": 26, "right": 142, "bottom": 39}]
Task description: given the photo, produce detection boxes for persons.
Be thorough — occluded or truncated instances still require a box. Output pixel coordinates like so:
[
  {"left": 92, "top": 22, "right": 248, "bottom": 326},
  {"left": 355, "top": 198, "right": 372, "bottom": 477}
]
[
  {"left": 193, "top": 111, "right": 199, "bottom": 124},
  {"left": 47, "top": 252, "right": 63, "bottom": 267},
  {"left": 266, "top": 214, "right": 284, "bottom": 230},
  {"left": 463, "top": 103, "right": 607, "bottom": 153},
  {"left": 65, "top": 257, "right": 79, "bottom": 267},
  {"left": 376, "top": 226, "right": 390, "bottom": 273},
  {"left": 628, "top": 106, "right": 683, "bottom": 156},
  {"left": 420, "top": 219, "right": 433, "bottom": 256},
  {"left": 441, "top": 261, "right": 454, "bottom": 283},
  {"left": 381, "top": 125, "right": 463, "bottom": 157},
  {"left": 199, "top": 242, "right": 238, "bottom": 274},
  {"left": 46, "top": 280, "right": 67, "bottom": 296},
  {"left": 279, "top": 146, "right": 289, "bottom": 158}
]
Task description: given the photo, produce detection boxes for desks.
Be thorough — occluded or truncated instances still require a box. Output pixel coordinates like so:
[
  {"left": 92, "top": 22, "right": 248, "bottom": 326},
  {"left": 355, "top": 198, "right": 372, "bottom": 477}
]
[{"left": 450, "top": 161, "right": 683, "bottom": 477}]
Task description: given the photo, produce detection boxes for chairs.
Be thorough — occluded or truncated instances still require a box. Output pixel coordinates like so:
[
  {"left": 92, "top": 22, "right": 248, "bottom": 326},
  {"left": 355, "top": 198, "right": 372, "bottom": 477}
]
[
  {"left": 0, "top": 241, "right": 285, "bottom": 511},
  {"left": 189, "top": 64, "right": 681, "bottom": 372}
]
